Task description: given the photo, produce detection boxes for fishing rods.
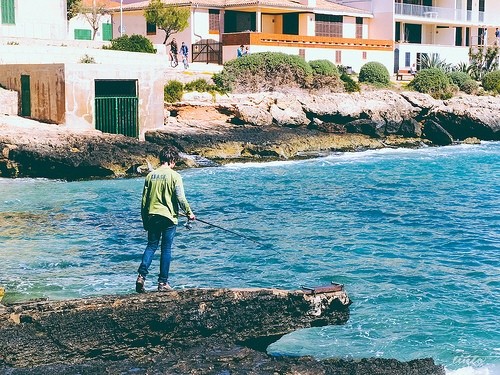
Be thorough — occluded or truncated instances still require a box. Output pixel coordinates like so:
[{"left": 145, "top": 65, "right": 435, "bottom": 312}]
[{"left": 179, "top": 210, "right": 282, "bottom": 256}]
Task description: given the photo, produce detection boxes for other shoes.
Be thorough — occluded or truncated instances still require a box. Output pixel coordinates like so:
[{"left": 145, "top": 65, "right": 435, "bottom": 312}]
[
  {"left": 136, "top": 276, "right": 144, "bottom": 293},
  {"left": 158, "top": 284, "right": 172, "bottom": 291}
]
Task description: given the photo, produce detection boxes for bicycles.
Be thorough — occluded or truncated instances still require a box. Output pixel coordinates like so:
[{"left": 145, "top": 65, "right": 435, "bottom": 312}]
[
  {"left": 181, "top": 53, "right": 189, "bottom": 69},
  {"left": 171, "top": 49, "right": 178, "bottom": 68}
]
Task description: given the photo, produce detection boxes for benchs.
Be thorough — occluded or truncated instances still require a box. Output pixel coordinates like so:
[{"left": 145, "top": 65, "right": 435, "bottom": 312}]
[{"left": 396, "top": 70, "right": 412, "bottom": 80}]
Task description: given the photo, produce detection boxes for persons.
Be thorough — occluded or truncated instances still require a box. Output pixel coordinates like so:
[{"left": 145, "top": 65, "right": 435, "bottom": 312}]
[
  {"left": 482, "top": 28, "right": 488, "bottom": 45},
  {"left": 179, "top": 42, "right": 190, "bottom": 69},
  {"left": 494, "top": 28, "right": 500, "bottom": 45},
  {"left": 165, "top": 37, "right": 179, "bottom": 65},
  {"left": 236, "top": 44, "right": 249, "bottom": 58},
  {"left": 412, "top": 63, "right": 417, "bottom": 78},
  {"left": 136, "top": 146, "right": 195, "bottom": 293}
]
